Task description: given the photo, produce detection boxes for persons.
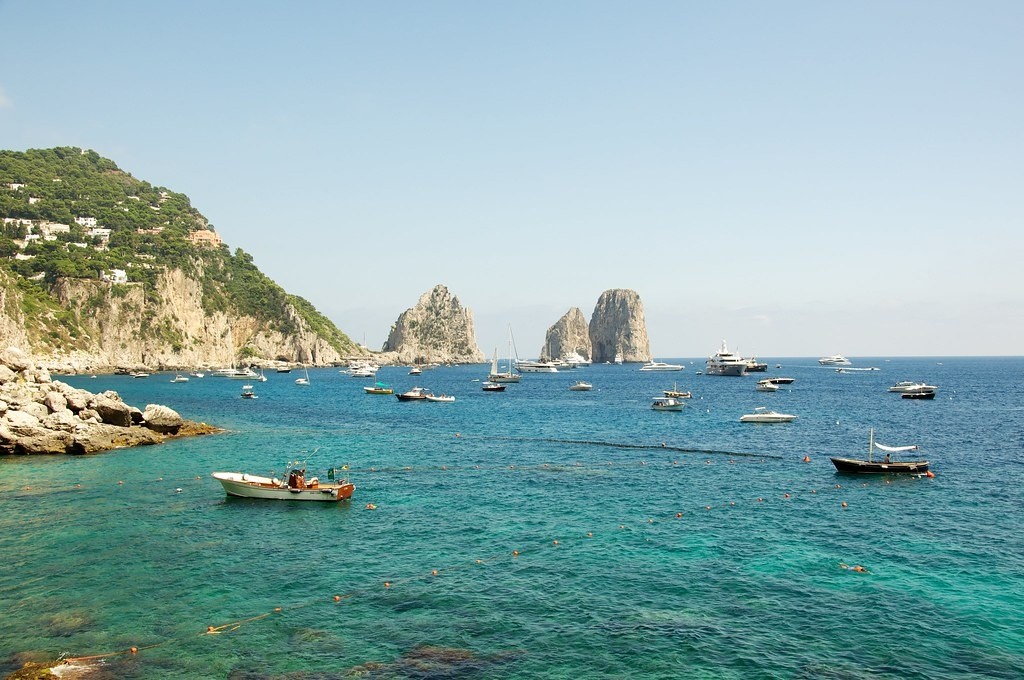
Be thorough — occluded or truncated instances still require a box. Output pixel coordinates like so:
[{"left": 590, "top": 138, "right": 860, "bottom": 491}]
[
  {"left": 653, "top": 400, "right": 664, "bottom": 406},
  {"left": 884, "top": 454, "right": 891, "bottom": 463}
]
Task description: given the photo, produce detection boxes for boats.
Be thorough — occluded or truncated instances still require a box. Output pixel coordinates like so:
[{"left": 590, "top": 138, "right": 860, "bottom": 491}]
[
  {"left": 817, "top": 353, "right": 848, "bottom": 366},
  {"left": 134, "top": 371, "right": 150, "bottom": 379},
  {"left": 755, "top": 381, "right": 780, "bottom": 392},
  {"left": 337, "top": 331, "right": 383, "bottom": 377},
  {"left": 210, "top": 367, "right": 268, "bottom": 383},
  {"left": 490, "top": 322, "right": 521, "bottom": 384},
  {"left": 395, "top": 385, "right": 434, "bottom": 402},
  {"left": 827, "top": 427, "right": 931, "bottom": 476},
  {"left": 650, "top": 398, "right": 685, "bottom": 411},
  {"left": 901, "top": 390, "right": 935, "bottom": 400},
  {"left": 425, "top": 393, "right": 456, "bottom": 403},
  {"left": 886, "top": 380, "right": 939, "bottom": 393},
  {"left": 508, "top": 322, "right": 593, "bottom": 374},
  {"left": 756, "top": 377, "right": 795, "bottom": 385},
  {"left": 169, "top": 374, "right": 190, "bottom": 383},
  {"left": 276, "top": 366, "right": 291, "bottom": 373},
  {"left": 364, "top": 386, "right": 394, "bottom": 394},
  {"left": 738, "top": 406, "right": 798, "bottom": 423},
  {"left": 482, "top": 384, "right": 506, "bottom": 392},
  {"left": 409, "top": 368, "right": 422, "bottom": 376},
  {"left": 569, "top": 380, "right": 593, "bottom": 391},
  {"left": 638, "top": 361, "right": 684, "bottom": 372},
  {"left": 745, "top": 353, "right": 767, "bottom": 372},
  {"left": 662, "top": 381, "right": 692, "bottom": 399},
  {"left": 189, "top": 369, "right": 205, "bottom": 378},
  {"left": 295, "top": 378, "right": 310, "bottom": 386},
  {"left": 210, "top": 444, "right": 357, "bottom": 502},
  {"left": 704, "top": 338, "right": 748, "bottom": 377},
  {"left": 240, "top": 390, "right": 254, "bottom": 398}
]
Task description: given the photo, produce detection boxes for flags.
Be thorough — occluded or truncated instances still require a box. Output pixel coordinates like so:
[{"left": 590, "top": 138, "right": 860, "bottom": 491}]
[
  {"left": 327, "top": 467, "right": 334, "bottom": 479},
  {"left": 341, "top": 464, "right": 349, "bottom": 470}
]
